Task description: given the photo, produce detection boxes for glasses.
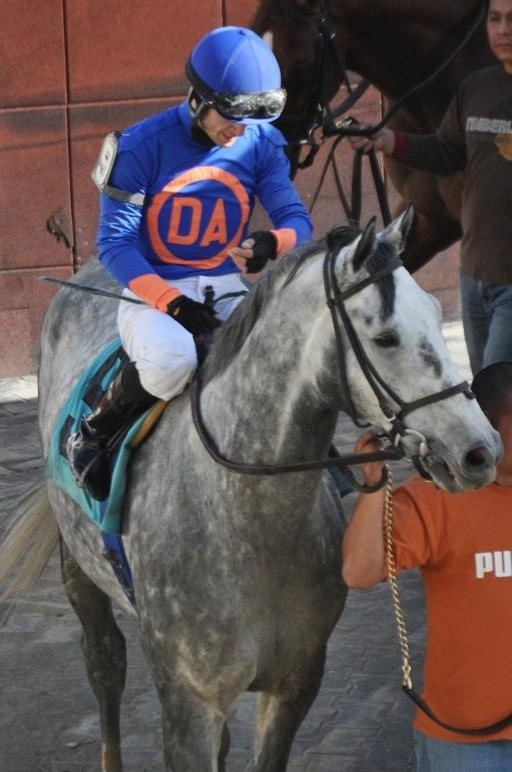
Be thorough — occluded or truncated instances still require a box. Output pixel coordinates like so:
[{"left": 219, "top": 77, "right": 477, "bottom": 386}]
[{"left": 211, "top": 89, "right": 287, "bottom": 121}]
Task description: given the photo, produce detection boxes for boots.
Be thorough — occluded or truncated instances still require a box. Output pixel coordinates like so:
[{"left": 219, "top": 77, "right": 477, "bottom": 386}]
[{"left": 65, "top": 368, "right": 143, "bottom": 501}]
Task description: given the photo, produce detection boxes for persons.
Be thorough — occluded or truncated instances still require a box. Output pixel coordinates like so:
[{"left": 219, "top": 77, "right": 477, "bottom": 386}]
[
  {"left": 343, "top": 0, "right": 512, "bottom": 378},
  {"left": 65, "top": 23, "right": 315, "bottom": 503},
  {"left": 338, "top": 360, "right": 512, "bottom": 771}
]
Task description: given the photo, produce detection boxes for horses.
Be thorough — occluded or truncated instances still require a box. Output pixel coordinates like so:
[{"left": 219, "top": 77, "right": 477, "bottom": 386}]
[
  {"left": 0, "top": 201, "right": 507, "bottom": 772},
  {"left": 248, "top": 0, "right": 502, "bottom": 276}
]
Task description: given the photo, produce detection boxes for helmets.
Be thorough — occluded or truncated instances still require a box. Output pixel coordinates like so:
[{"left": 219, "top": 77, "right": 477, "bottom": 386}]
[{"left": 184, "top": 26, "right": 282, "bottom": 124}]
